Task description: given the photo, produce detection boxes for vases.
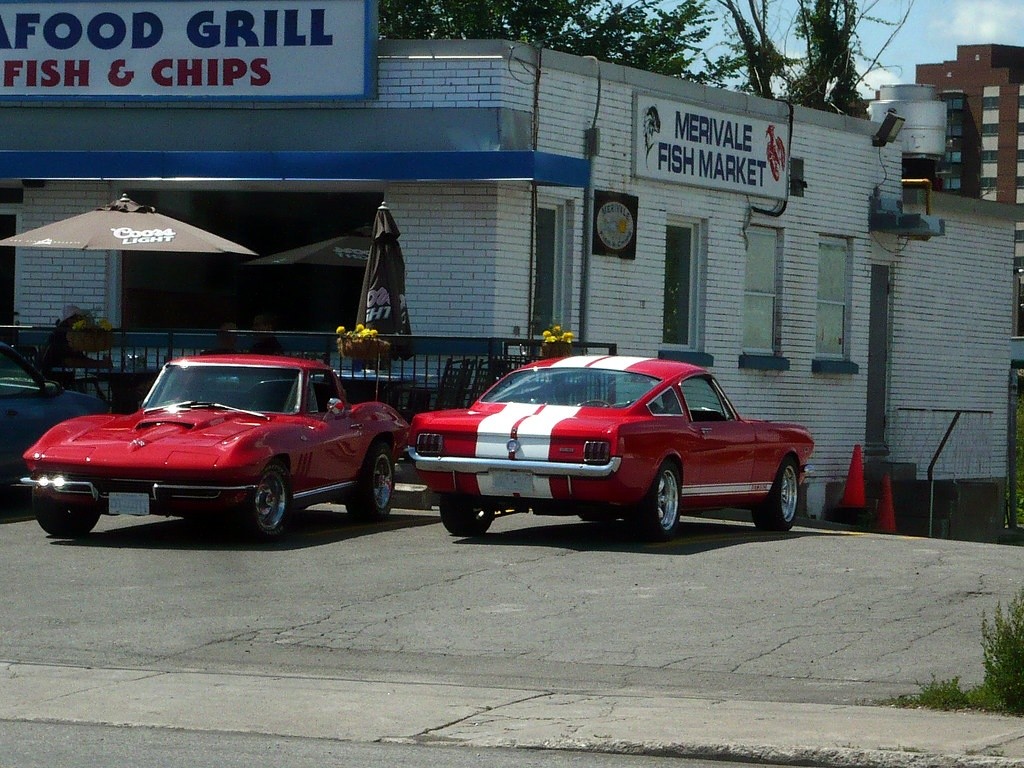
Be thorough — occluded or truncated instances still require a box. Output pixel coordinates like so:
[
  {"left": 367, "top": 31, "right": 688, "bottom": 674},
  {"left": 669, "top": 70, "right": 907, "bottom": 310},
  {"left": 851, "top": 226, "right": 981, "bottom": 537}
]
[
  {"left": 542, "top": 342, "right": 572, "bottom": 356},
  {"left": 67, "top": 331, "right": 115, "bottom": 351},
  {"left": 337, "top": 337, "right": 390, "bottom": 361}
]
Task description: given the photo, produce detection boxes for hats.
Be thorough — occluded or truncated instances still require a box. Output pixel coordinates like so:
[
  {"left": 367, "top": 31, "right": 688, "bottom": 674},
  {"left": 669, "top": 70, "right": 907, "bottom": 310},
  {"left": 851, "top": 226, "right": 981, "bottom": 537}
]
[{"left": 57, "top": 305, "right": 91, "bottom": 324}]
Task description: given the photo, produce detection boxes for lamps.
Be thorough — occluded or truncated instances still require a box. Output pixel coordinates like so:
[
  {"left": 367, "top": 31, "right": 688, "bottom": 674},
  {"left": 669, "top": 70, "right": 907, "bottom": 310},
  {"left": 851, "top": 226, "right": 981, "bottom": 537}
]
[
  {"left": 23, "top": 180, "right": 44, "bottom": 187},
  {"left": 872, "top": 113, "right": 905, "bottom": 146}
]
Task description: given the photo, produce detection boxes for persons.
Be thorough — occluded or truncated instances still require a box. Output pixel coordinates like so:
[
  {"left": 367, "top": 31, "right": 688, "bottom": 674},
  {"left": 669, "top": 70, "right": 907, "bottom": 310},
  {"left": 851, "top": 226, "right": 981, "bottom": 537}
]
[
  {"left": 247, "top": 310, "right": 286, "bottom": 356},
  {"left": 201, "top": 321, "right": 242, "bottom": 357},
  {"left": 41, "top": 304, "right": 114, "bottom": 390}
]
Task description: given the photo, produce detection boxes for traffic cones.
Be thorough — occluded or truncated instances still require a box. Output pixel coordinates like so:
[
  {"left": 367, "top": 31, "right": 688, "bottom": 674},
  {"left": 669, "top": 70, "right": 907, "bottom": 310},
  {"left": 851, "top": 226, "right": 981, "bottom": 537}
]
[
  {"left": 870, "top": 473, "right": 900, "bottom": 534},
  {"left": 841, "top": 444, "right": 868, "bottom": 509}
]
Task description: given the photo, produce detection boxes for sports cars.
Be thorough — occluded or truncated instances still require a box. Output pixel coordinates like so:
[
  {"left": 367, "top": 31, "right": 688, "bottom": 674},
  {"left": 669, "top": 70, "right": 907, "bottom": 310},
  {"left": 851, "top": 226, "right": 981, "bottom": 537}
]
[
  {"left": 18, "top": 353, "right": 411, "bottom": 541},
  {"left": 406, "top": 354, "right": 818, "bottom": 539}
]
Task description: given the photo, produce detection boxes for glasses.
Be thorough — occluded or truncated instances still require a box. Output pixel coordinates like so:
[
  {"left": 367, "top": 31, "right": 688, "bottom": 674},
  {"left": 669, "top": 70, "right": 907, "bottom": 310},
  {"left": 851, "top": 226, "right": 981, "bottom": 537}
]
[{"left": 76, "top": 317, "right": 84, "bottom": 321}]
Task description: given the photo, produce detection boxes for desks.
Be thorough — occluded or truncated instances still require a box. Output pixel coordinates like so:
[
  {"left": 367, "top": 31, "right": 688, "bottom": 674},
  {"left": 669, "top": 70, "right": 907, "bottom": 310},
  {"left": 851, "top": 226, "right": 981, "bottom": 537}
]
[{"left": 315, "top": 371, "right": 416, "bottom": 410}]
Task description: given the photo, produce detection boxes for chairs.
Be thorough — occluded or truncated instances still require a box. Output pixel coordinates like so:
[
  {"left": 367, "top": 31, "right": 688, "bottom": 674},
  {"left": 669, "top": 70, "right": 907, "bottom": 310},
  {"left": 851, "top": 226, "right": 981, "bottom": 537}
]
[{"left": 411, "top": 357, "right": 521, "bottom": 410}]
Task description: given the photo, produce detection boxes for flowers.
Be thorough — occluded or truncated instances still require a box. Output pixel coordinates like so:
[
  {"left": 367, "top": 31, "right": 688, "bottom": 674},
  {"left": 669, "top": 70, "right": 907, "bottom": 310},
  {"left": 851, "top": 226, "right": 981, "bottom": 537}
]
[
  {"left": 336, "top": 323, "right": 377, "bottom": 338},
  {"left": 64, "top": 306, "right": 113, "bottom": 331},
  {"left": 544, "top": 325, "right": 573, "bottom": 343}
]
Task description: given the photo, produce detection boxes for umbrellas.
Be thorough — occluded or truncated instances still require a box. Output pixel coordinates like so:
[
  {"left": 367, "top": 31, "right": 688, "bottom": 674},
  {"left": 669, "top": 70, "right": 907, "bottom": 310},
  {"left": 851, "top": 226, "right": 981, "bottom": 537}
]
[
  {"left": 352, "top": 200, "right": 419, "bottom": 373},
  {"left": 243, "top": 225, "right": 378, "bottom": 332},
  {"left": 0, "top": 189, "right": 260, "bottom": 367}
]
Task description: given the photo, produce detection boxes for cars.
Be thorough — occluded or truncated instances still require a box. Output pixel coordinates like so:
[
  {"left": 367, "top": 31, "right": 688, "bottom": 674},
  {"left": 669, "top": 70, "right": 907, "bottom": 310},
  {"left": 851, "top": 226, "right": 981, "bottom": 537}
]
[{"left": 0, "top": 341, "right": 109, "bottom": 489}]
